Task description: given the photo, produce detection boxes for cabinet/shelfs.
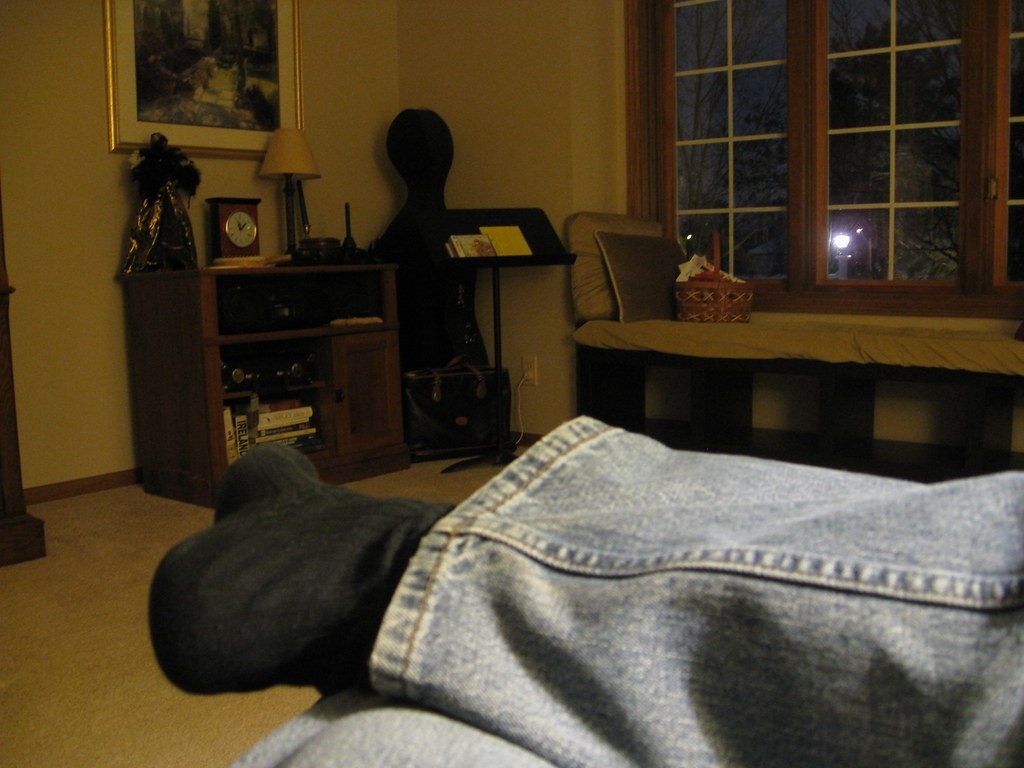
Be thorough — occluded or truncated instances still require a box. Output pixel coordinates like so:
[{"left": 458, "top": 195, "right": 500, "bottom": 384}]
[{"left": 115, "top": 264, "right": 410, "bottom": 509}]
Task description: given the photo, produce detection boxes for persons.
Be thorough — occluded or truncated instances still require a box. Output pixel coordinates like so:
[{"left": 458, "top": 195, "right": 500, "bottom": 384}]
[{"left": 149, "top": 412, "right": 1023, "bottom": 767}]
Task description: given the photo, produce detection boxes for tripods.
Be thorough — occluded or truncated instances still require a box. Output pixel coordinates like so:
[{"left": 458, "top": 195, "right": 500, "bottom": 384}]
[{"left": 427, "top": 207, "right": 577, "bottom": 475}]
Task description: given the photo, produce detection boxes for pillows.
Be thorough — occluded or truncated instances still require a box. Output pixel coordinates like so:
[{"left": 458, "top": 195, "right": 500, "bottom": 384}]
[{"left": 568, "top": 212, "right": 687, "bottom": 324}]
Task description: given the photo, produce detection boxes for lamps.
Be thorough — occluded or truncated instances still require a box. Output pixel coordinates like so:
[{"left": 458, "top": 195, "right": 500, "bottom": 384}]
[{"left": 259, "top": 128, "right": 321, "bottom": 260}]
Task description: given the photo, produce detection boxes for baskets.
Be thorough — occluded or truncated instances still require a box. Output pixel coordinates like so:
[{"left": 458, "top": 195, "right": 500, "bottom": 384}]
[{"left": 673, "top": 230, "right": 753, "bottom": 323}]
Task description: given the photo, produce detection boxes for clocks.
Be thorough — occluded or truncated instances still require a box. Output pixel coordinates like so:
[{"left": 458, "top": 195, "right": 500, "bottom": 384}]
[{"left": 205, "top": 197, "right": 261, "bottom": 257}]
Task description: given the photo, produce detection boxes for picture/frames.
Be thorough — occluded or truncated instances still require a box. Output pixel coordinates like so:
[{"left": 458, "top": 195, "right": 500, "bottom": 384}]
[{"left": 102, "top": 0, "right": 304, "bottom": 160}]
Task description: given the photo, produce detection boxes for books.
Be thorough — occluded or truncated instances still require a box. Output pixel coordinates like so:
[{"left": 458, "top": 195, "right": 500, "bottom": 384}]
[{"left": 224, "top": 392, "right": 318, "bottom": 465}]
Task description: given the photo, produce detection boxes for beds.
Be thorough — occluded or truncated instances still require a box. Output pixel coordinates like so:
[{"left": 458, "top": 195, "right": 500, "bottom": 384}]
[{"left": 567, "top": 214, "right": 1024, "bottom": 472}]
